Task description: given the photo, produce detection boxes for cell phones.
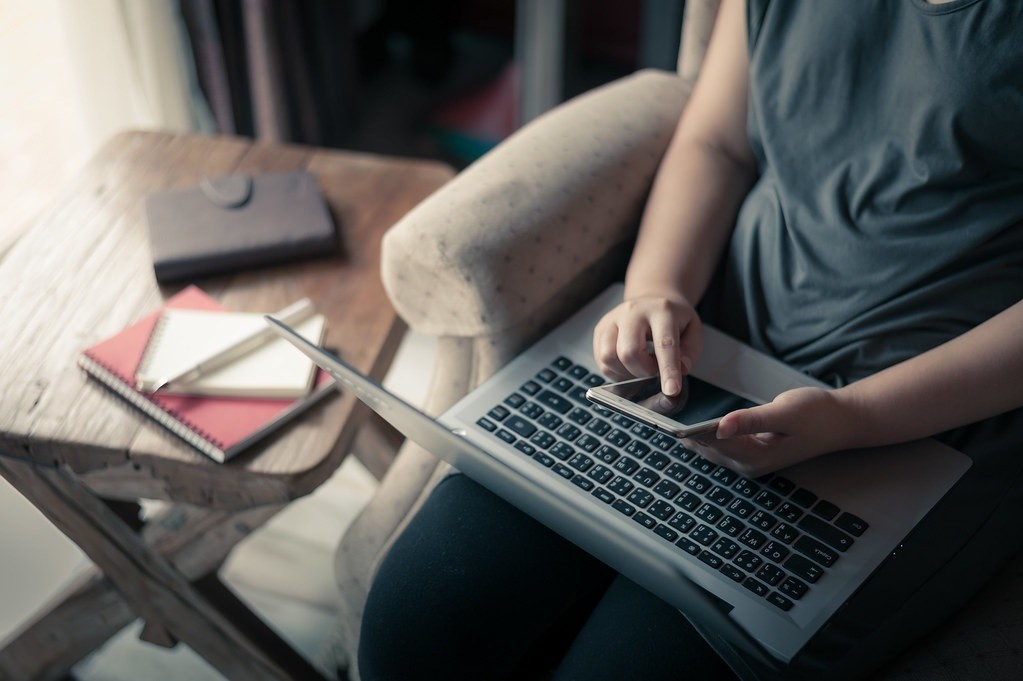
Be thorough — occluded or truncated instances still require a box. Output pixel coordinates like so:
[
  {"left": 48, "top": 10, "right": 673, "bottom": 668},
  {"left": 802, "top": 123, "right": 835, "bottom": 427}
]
[{"left": 584, "top": 374, "right": 766, "bottom": 441}]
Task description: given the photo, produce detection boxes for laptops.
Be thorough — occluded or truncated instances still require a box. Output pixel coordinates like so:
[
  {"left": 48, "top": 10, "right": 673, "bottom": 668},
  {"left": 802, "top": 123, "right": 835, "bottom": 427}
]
[{"left": 265, "top": 278, "right": 972, "bottom": 680}]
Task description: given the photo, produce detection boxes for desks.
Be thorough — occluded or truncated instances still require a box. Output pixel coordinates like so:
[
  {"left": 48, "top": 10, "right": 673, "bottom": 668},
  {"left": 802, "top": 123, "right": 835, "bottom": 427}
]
[{"left": 0, "top": 130, "right": 460, "bottom": 681}]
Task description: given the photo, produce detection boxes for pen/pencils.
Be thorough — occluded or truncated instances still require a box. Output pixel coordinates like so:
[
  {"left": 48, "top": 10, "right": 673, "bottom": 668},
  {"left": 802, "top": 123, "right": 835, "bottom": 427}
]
[{"left": 150, "top": 295, "right": 316, "bottom": 397}]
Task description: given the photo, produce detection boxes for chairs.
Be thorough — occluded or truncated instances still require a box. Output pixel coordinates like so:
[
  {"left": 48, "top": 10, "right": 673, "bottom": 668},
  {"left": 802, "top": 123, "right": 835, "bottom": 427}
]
[{"left": 329, "top": 1, "right": 717, "bottom": 681}]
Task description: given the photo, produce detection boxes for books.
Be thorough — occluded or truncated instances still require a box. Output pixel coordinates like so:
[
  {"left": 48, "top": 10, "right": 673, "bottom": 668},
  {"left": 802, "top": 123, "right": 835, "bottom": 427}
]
[{"left": 75, "top": 284, "right": 342, "bottom": 464}]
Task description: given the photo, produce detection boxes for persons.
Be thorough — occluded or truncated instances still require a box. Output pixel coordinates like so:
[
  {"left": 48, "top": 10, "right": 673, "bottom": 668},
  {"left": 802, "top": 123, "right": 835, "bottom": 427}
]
[{"left": 357, "top": 0, "right": 1023, "bottom": 681}]
